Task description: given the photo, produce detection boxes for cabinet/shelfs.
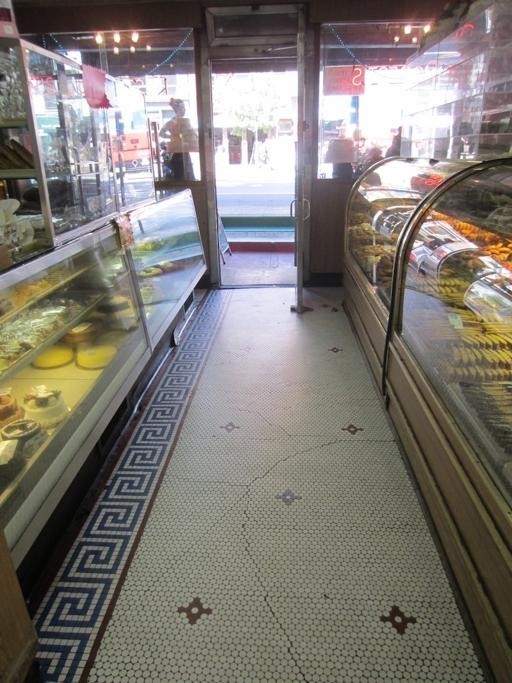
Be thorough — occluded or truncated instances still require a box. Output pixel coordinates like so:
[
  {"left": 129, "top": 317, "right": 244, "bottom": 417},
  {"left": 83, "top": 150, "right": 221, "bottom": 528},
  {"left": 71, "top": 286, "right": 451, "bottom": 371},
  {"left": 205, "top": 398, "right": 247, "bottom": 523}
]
[
  {"left": 1, "top": 38, "right": 207, "bottom": 569},
  {"left": 340, "top": 156, "right": 511, "bottom": 678}
]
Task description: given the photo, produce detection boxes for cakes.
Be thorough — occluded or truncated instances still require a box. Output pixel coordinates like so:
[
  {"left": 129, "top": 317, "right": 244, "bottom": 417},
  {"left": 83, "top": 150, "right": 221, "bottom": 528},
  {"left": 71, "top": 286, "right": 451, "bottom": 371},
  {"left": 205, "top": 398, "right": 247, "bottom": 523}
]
[
  {"left": 32, "top": 261, "right": 179, "bottom": 369},
  {"left": 0, "top": 382, "right": 70, "bottom": 496}
]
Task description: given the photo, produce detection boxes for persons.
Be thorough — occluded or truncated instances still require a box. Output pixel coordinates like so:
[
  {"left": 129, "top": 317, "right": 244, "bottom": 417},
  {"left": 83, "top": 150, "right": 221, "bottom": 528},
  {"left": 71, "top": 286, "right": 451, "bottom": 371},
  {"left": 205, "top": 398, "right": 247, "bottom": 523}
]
[{"left": 159, "top": 98, "right": 195, "bottom": 181}]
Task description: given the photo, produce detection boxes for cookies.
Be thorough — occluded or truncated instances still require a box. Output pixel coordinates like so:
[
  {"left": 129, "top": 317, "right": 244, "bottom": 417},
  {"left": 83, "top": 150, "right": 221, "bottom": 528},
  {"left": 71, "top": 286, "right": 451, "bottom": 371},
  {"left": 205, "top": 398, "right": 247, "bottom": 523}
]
[{"left": 405, "top": 210, "right": 512, "bottom": 456}]
